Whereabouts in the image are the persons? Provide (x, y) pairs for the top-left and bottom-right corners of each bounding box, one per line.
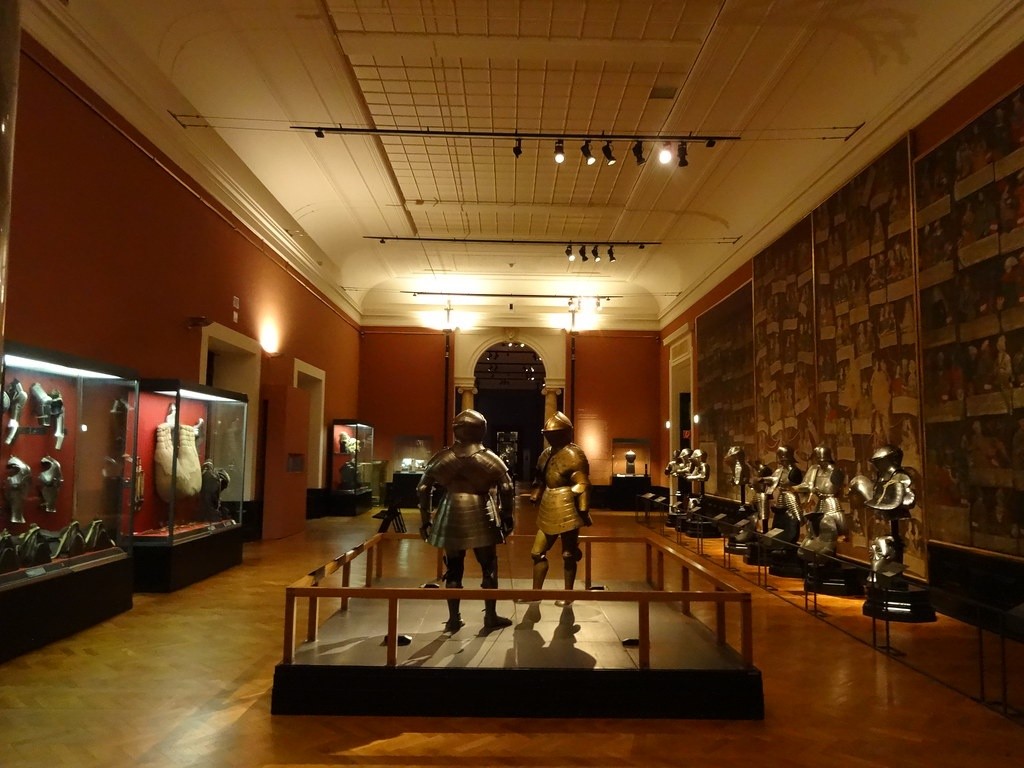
(725, 446), (750, 484)
(677, 448), (695, 480)
(686, 449), (710, 481)
(514, 413), (592, 603)
(666, 450), (683, 475)
(415, 410), (517, 634)
(769, 447), (804, 510)
(865, 444), (917, 514)
(793, 445), (849, 555)
